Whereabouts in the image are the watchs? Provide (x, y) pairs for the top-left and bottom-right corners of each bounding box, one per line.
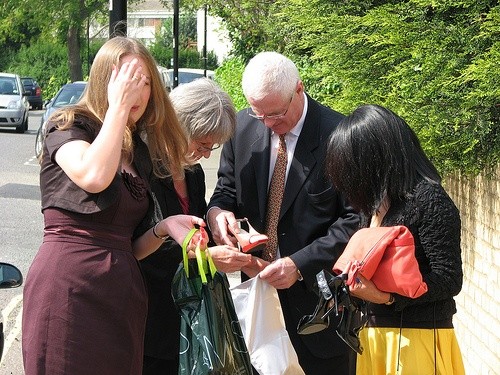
(385, 293), (394, 306)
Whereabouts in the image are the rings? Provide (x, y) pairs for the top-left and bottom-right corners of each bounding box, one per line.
(133, 76), (139, 82)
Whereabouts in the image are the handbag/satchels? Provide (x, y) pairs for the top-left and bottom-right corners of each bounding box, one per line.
(332, 226), (428, 298)
(229, 274), (307, 375)
(170, 227), (253, 375)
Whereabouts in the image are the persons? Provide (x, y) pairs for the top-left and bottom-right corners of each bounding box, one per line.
(21, 35), (209, 375)
(202, 52), (357, 375)
(139, 78), (270, 375)
(325, 104), (465, 375)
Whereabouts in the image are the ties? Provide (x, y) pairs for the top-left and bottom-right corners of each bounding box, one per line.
(261, 132), (287, 263)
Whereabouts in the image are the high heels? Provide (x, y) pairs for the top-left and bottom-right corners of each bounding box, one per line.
(321, 285), (366, 356)
(296, 269), (342, 335)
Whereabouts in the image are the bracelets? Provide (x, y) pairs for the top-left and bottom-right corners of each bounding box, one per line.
(152, 222), (169, 240)
(296, 268), (302, 277)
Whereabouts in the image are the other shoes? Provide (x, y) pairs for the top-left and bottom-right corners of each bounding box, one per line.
(227, 218), (267, 253)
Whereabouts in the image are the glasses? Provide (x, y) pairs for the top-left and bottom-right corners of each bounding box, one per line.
(193, 140), (221, 151)
(247, 88), (295, 119)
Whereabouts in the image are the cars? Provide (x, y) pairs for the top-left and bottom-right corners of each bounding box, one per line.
(0, 72), (30, 133)
(34, 81), (88, 160)
(164, 66), (215, 92)
(20, 76), (43, 109)
